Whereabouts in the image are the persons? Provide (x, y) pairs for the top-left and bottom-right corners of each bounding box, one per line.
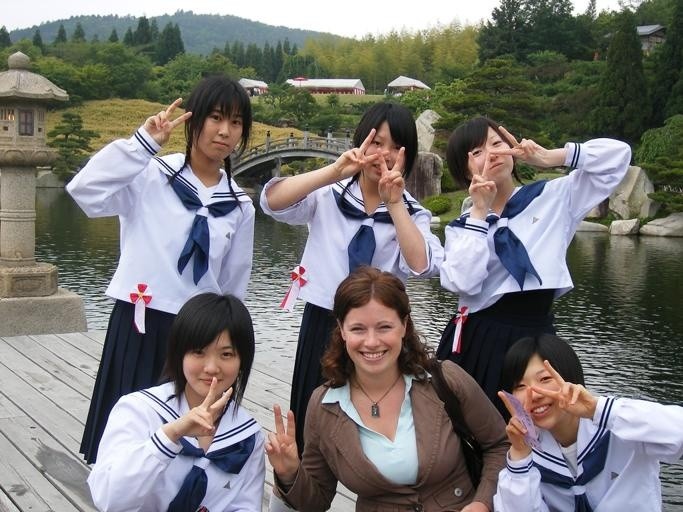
(490, 332), (683, 512)
(262, 264), (511, 512)
(430, 116), (635, 422)
(256, 100), (447, 512)
(62, 75), (256, 464)
(85, 291), (266, 512)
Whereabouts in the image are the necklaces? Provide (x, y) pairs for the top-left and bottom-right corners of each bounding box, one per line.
(352, 372), (402, 419)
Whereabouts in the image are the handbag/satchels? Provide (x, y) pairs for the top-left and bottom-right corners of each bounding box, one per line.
(444, 398), (483, 488)
